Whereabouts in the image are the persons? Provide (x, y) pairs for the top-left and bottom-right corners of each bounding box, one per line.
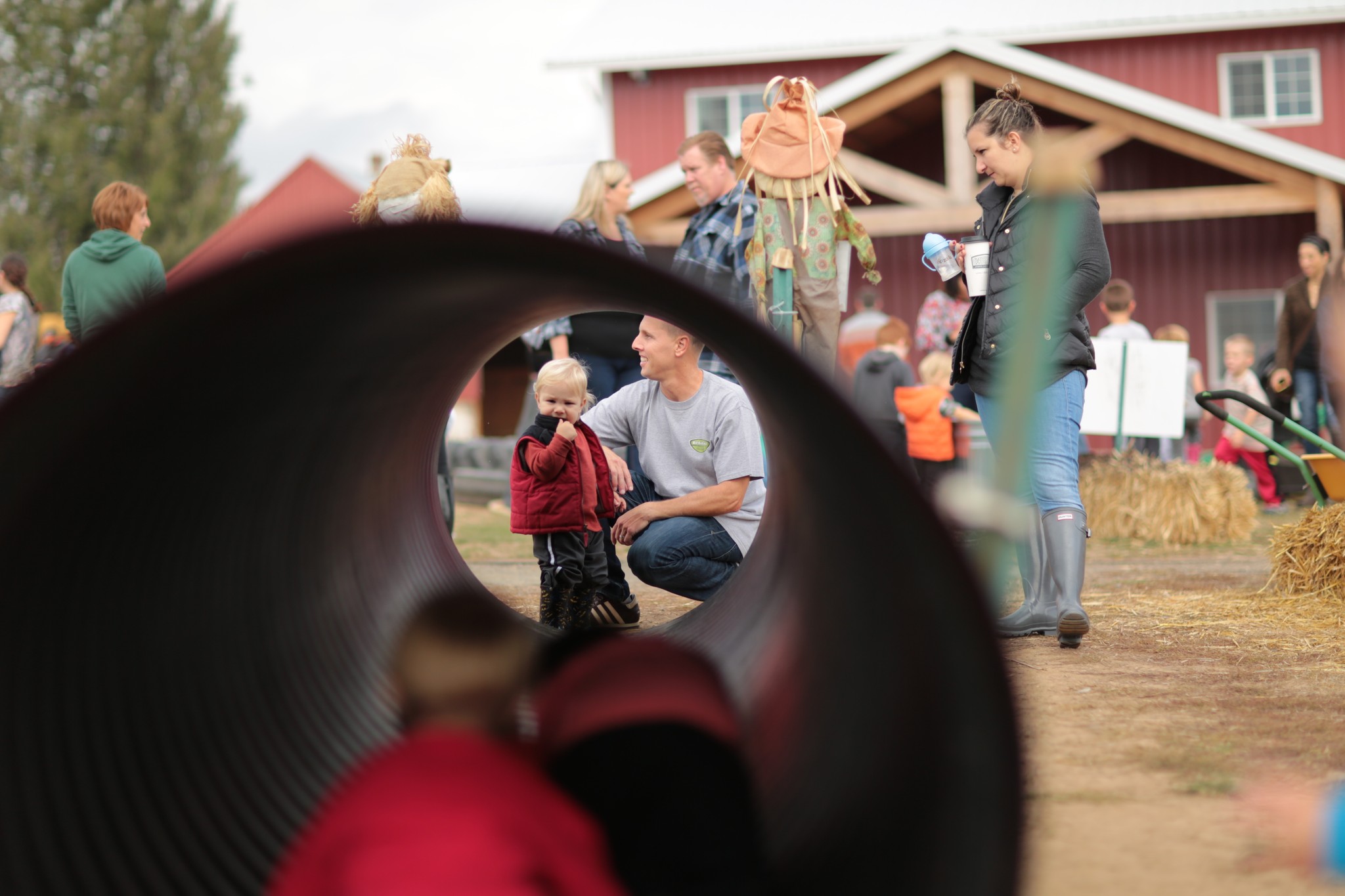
(731, 73), (885, 383)
(578, 309), (770, 632)
(59, 182), (171, 352)
(665, 134), (764, 316)
(532, 156), (659, 412)
(938, 72), (1112, 648)
(921, 266), (973, 365)
(1090, 273), (1151, 463)
(265, 578), (629, 896)
(507, 356), (626, 634)
(1267, 234), (1344, 502)
(1151, 323), (1216, 447)
(1, 252), (40, 406)
(890, 353), (987, 489)
(1205, 332), (1280, 515)
(850, 315), (915, 466)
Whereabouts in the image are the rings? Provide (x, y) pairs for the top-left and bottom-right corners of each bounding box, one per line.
(619, 532), (627, 538)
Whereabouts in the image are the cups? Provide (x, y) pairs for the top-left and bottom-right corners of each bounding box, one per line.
(961, 236), (994, 299)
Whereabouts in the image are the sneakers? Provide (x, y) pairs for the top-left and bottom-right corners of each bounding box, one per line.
(586, 593), (640, 627)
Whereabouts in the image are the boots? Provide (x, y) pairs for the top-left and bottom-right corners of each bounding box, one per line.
(990, 506), (1060, 636)
(1041, 506), (1091, 648)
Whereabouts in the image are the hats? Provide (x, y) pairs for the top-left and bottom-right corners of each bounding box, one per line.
(740, 76), (848, 180)
(893, 386), (945, 421)
(79, 227), (139, 262)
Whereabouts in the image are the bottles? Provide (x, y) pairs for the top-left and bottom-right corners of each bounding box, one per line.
(920, 232), (961, 282)
